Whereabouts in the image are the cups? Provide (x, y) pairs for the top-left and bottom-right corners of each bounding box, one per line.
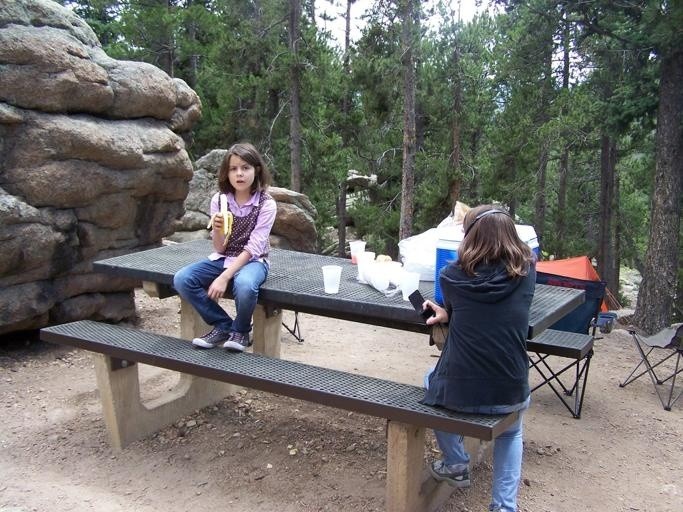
(400, 272), (419, 300)
(347, 239), (366, 265)
(357, 252), (408, 297)
(321, 265), (343, 294)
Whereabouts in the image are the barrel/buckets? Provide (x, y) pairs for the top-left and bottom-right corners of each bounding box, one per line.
(598, 315), (614, 334)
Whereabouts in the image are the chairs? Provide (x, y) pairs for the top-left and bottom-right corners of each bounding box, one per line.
(618, 319), (683, 411)
(530, 267), (608, 420)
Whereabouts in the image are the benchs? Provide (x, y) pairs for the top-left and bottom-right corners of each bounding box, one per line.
(40, 318), (519, 511)
(252, 301), (594, 419)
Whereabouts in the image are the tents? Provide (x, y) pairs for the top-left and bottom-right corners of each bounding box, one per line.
(528, 253), (609, 315)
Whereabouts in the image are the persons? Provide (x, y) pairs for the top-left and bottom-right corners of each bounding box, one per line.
(415, 205), (539, 512)
(173, 142), (279, 351)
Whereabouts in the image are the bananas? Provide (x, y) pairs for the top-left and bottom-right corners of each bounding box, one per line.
(206, 194), (233, 247)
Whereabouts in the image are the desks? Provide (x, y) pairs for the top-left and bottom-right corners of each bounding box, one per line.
(91, 239), (584, 451)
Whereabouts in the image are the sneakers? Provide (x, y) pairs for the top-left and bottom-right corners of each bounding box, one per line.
(223, 332), (248, 352)
(431, 460), (470, 488)
(193, 328), (227, 349)
